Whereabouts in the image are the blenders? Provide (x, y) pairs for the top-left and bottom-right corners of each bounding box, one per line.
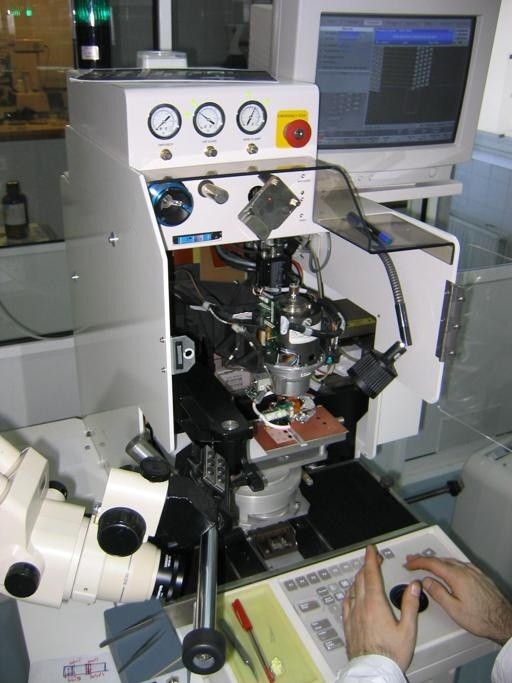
(10, 39), (50, 114)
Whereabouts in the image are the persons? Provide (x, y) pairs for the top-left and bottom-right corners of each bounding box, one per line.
(335, 542), (512, 683)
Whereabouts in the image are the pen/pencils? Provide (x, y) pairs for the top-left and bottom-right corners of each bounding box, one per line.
(231, 598), (275, 683)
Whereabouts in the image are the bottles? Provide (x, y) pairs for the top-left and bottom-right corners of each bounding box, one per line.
(74, 0), (111, 68)
(1, 180), (29, 241)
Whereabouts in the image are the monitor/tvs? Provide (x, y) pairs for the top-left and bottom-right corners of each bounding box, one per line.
(247, 0), (503, 191)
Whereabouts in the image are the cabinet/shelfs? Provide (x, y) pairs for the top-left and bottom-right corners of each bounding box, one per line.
(0, 0), (172, 363)
